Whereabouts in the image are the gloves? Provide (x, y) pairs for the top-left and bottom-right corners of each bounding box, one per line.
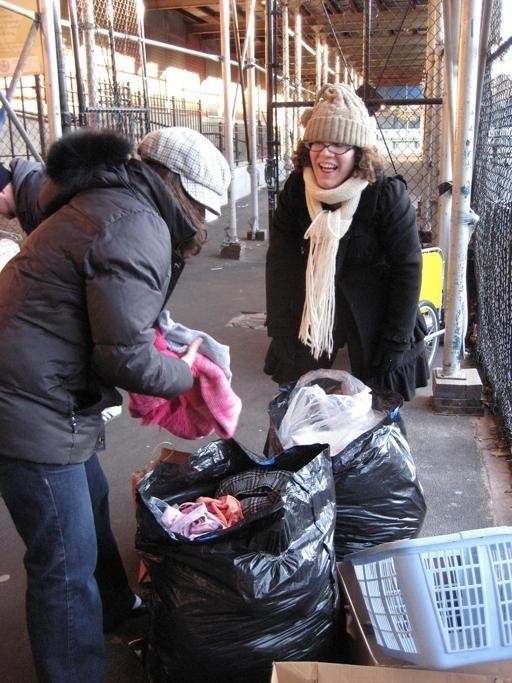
(372, 344), (403, 374)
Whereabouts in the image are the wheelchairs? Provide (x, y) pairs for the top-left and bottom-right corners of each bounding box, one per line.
(416, 248), (444, 367)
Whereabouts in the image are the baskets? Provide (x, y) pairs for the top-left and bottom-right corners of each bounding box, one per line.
(343, 525), (512, 670)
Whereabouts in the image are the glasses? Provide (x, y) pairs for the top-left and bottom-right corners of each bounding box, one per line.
(308, 141), (354, 155)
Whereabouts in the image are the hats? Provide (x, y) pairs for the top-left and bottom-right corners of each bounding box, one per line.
(301, 82), (371, 153)
(136, 126), (231, 217)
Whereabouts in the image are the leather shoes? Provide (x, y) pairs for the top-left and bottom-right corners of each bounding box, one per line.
(104, 592), (144, 621)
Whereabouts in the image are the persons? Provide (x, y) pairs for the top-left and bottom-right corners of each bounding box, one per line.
(260, 82), (432, 417)
(2, 154), (60, 239)
(1, 123), (235, 682)
